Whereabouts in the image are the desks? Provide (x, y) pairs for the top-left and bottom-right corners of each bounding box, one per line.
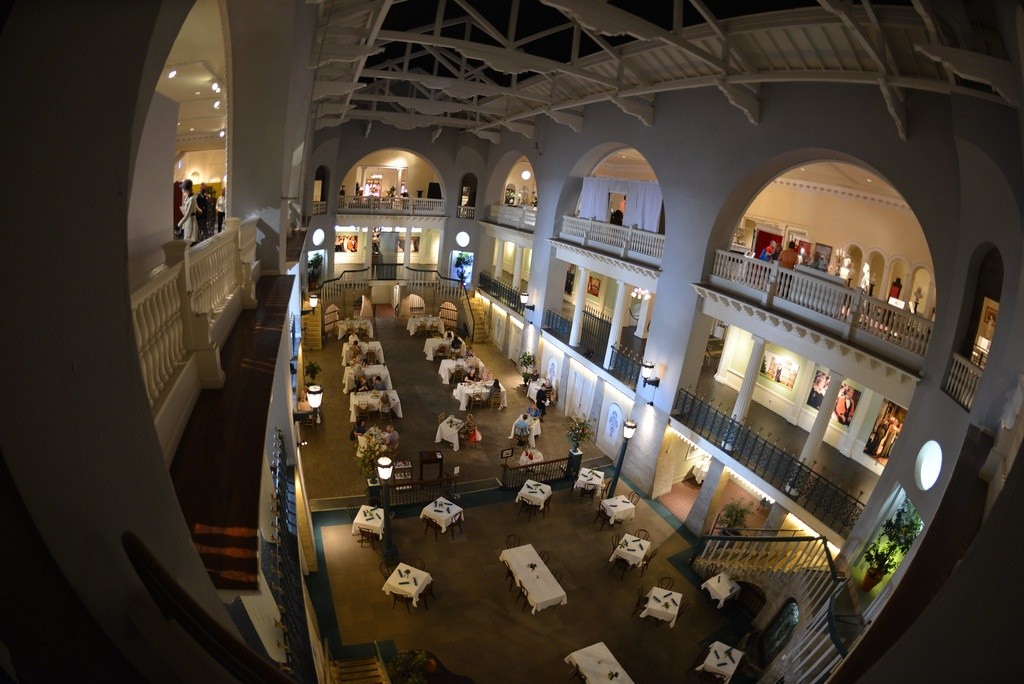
(565, 642), (634, 684)
(639, 587), (683, 629)
(499, 544), (567, 615)
(526, 382), (550, 406)
(456, 381), (507, 411)
(438, 357), (485, 385)
(338, 320), (373, 340)
(573, 468), (604, 498)
(695, 641), (745, 684)
(701, 571), (742, 609)
(516, 479), (552, 511)
(508, 414), (541, 448)
(407, 317), (444, 336)
(352, 505), (384, 540)
(597, 495), (635, 524)
(423, 337), (466, 361)
(519, 449), (544, 471)
(382, 563), (432, 608)
(435, 415), (482, 451)
(692, 460), (709, 487)
(609, 533), (651, 571)
(420, 496), (464, 533)
(342, 341), (402, 457)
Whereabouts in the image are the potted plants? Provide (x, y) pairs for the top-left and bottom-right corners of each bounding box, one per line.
(862, 495), (924, 591)
(455, 252), (474, 289)
(303, 361), (323, 394)
(518, 352), (535, 382)
(719, 500), (756, 538)
(308, 253), (324, 290)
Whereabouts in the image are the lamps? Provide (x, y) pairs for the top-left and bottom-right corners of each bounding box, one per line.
(520, 293), (535, 312)
(977, 336), (992, 367)
(292, 384), (323, 425)
(641, 361), (660, 388)
(630, 288), (651, 301)
(295, 439), (308, 448)
(301, 294), (318, 316)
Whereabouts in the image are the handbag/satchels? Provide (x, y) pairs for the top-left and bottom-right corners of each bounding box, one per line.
(475, 428), (482, 441)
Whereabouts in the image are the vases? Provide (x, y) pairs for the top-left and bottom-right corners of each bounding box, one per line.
(572, 442), (580, 452)
(370, 470), (377, 484)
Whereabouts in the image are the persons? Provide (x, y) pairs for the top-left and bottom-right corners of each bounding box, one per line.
(463, 367), (476, 383)
(382, 425), (400, 450)
(444, 327), (477, 357)
(765, 239), (798, 299)
(300, 386), (308, 402)
(775, 364), (782, 382)
(350, 419), (365, 441)
(515, 370), (548, 442)
(344, 330), (386, 391)
(484, 378), (500, 408)
(835, 383), (858, 426)
(177, 180), (225, 248)
(865, 414), (901, 458)
(531, 197), (538, 207)
(459, 413), (475, 439)
(334, 236), (358, 252)
(807, 370), (830, 410)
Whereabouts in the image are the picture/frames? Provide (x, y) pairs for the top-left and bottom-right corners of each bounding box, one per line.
(984, 307), (1000, 327)
(814, 241), (833, 271)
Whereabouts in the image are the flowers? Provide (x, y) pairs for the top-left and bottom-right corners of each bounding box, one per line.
(353, 435), (388, 479)
(564, 413), (597, 444)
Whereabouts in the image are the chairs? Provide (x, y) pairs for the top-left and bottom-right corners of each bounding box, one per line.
(346, 464), (747, 684)
(347, 319), (391, 426)
(417, 321), (554, 447)
(388, 438), (401, 460)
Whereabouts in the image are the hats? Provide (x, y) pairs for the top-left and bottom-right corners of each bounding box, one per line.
(179, 179), (193, 191)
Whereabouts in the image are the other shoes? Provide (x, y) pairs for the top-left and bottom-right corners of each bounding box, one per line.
(540, 418), (544, 423)
